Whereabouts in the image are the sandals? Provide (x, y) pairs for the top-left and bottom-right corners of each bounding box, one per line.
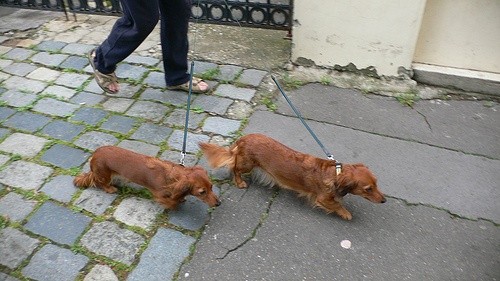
(89, 48), (120, 94)
(167, 76), (209, 93)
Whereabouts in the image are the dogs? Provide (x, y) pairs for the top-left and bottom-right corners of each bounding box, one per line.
(74, 145), (222, 211)
(198, 133), (386, 221)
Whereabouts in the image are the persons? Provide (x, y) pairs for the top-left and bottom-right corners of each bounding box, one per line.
(87, 0), (210, 94)
